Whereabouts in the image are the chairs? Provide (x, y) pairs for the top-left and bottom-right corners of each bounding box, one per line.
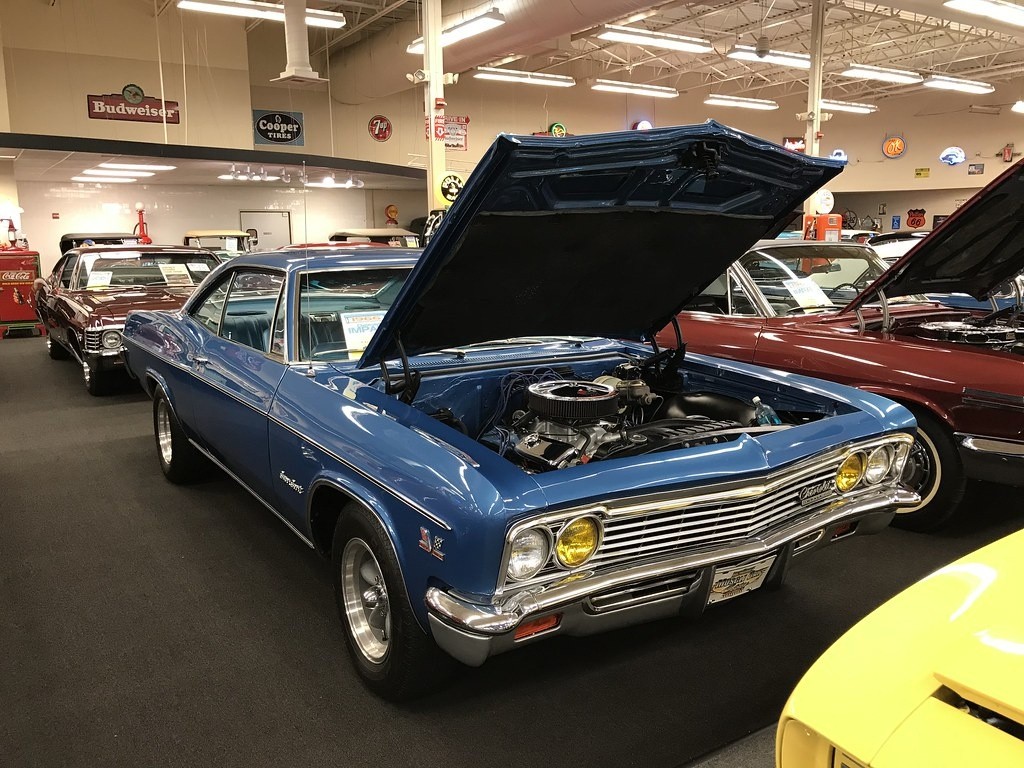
(262, 320), (319, 359)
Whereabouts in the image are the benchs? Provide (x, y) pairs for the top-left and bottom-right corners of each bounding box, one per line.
(223, 304), (389, 360)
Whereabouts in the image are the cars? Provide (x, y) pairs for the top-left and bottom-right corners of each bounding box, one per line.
(119, 120), (923, 710)
(776, 530), (1024, 768)
(32, 228), (1020, 398)
(649, 159), (1024, 533)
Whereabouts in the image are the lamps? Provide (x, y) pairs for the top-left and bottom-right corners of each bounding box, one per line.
(279, 168), (291, 183)
(245, 167), (255, 180)
(472, 66), (577, 88)
(406, 68), (430, 84)
(968, 104), (1001, 115)
(257, 165), (268, 182)
(345, 176), (364, 188)
(405, 8), (505, 54)
(838, 62), (924, 85)
(726, 45), (812, 68)
(443, 73), (459, 85)
(298, 172), (307, 184)
(924, 75), (996, 95)
(943, 0), (1024, 27)
(175, 0), (346, 29)
(230, 164), (241, 179)
(821, 98), (880, 114)
(703, 94), (779, 110)
(588, 77), (679, 99)
(598, 24), (715, 54)
